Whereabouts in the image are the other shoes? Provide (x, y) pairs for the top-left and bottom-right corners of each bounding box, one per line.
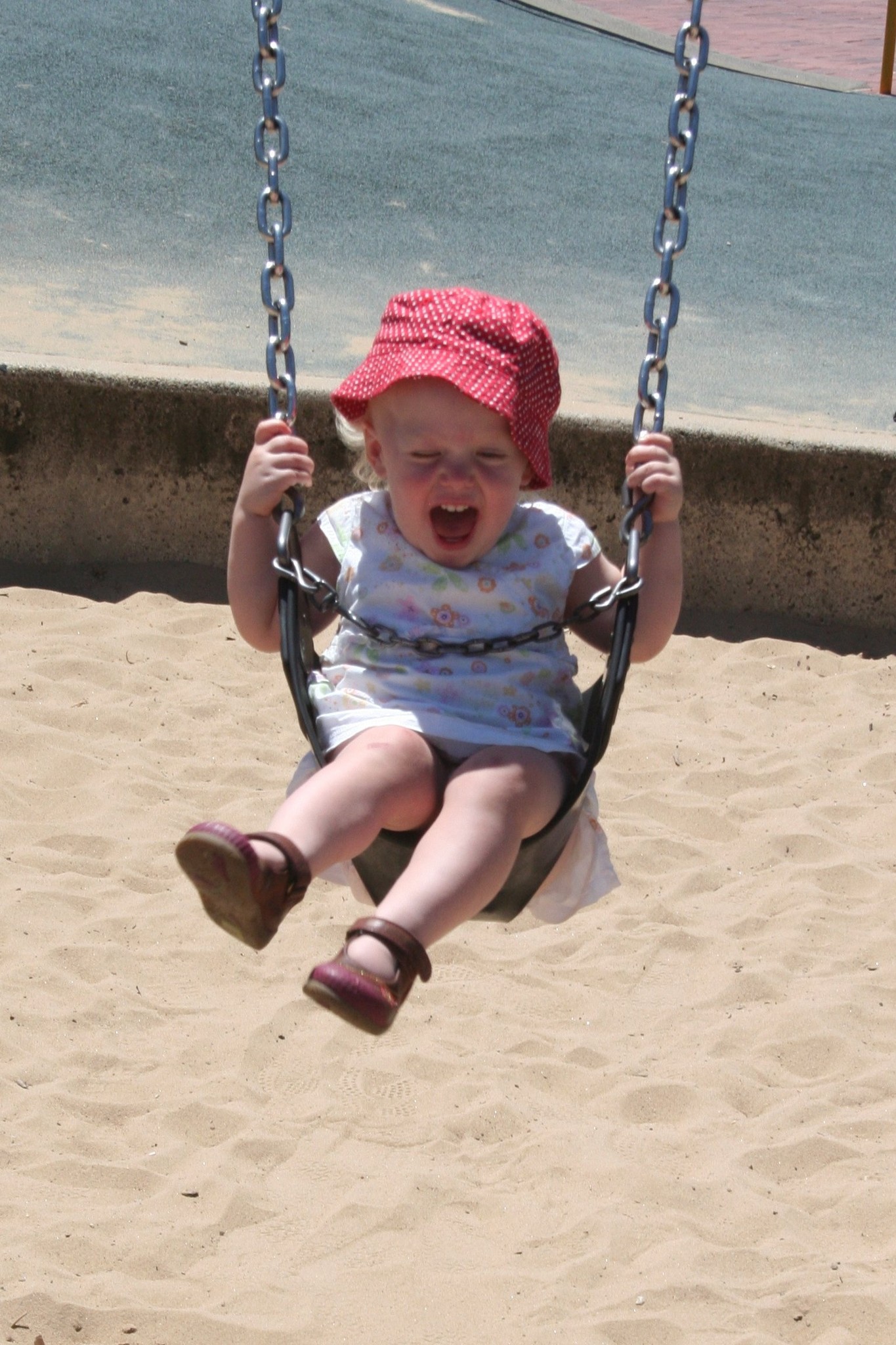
(303, 917), (431, 1036)
(177, 822), (311, 948)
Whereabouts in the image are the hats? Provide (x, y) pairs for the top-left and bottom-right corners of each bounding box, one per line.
(331, 289), (561, 491)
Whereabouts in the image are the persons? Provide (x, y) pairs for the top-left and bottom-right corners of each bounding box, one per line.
(173, 280), (695, 1035)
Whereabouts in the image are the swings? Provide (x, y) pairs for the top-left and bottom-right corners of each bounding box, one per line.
(250, 0), (710, 924)
